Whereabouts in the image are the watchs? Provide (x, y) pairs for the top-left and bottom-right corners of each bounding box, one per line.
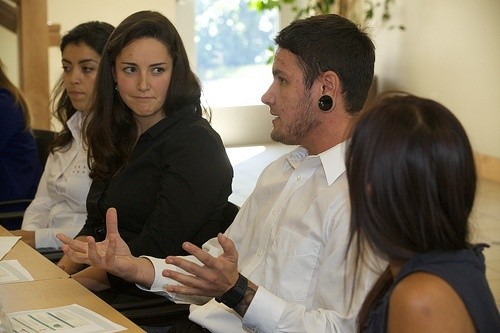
(215, 273), (248, 308)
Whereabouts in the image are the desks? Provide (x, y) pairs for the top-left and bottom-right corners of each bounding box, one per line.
(0, 225), (148, 333)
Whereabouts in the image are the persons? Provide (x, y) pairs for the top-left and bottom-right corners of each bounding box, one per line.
(56, 14), (375, 333)
(56, 10), (233, 333)
(10, 22), (115, 250)
(0, 60), (39, 212)
(345, 96), (500, 333)
